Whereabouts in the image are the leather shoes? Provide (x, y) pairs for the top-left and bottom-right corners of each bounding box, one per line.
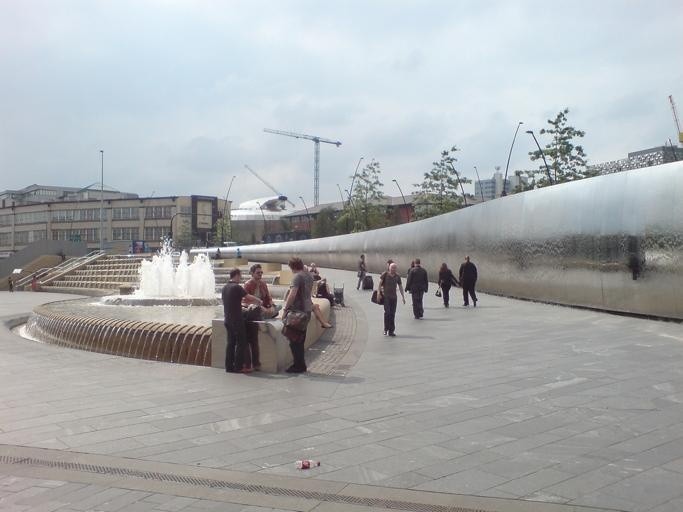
(384, 331), (387, 335)
(473, 299), (477, 307)
(463, 302), (469, 306)
(322, 324), (333, 328)
(286, 364), (306, 372)
(389, 333), (396, 336)
(226, 367), (261, 373)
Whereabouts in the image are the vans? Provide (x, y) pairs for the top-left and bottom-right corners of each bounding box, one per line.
(0, 252), (15, 258)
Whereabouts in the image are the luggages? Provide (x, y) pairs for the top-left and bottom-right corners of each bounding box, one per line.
(362, 276), (373, 290)
(333, 283), (344, 304)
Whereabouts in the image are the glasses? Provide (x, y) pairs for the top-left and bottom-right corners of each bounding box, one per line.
(257, 272), (263, 274)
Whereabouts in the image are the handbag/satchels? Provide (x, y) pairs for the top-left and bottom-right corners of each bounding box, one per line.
(357, 271), (365, 277)
(435, 290), (441, 297)
(371, 290), (385, 305)
(282, 309), (309, 333)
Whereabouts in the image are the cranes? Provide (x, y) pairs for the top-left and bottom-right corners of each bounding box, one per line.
(261, 127), (344, 204)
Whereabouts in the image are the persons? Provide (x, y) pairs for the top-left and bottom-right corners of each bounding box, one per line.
(385, 260), (393, 271)
(242, 263), (283, 320)
(8, 277), (13, 292)
(375, 262), (406, 338)
(213, 248), (223, 259)
(315, 278), (335, 306)
(308, 262), (321, 281)
(127, 244), (131, 253)
(457, 254), (478, 309)
(403, 257), (428, 321)
(284, 285), (333, 331)
(220, 267), (263, 373)
(31, 275), (37, 291)
(271, 275), (280, 285)
(355, 254), (368, 290)
(235, 248), (241, 259)
(437, 262), (458, 309)
(406, 260), (414, 277)
(279, 256), (313, 376)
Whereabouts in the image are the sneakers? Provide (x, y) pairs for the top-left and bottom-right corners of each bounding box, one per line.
(275, 305), (282, 311)
(415, 316), (424, 320)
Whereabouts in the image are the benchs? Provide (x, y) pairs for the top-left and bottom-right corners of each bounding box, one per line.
(211, 294), (330, 374)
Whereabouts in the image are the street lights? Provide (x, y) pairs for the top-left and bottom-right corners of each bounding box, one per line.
(444, 161), (467, 207)
(170, 212), (188, 247)
(99, 149), (105, 251)
(524, 129), (553, 186)
(221, 157), (369, 242)
(500, 120), (525, 197)
(391, 179), (410, 225)
(473, 166), (484, 202)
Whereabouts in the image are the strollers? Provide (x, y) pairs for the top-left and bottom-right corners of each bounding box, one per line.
(331, 283), (347, 308)
